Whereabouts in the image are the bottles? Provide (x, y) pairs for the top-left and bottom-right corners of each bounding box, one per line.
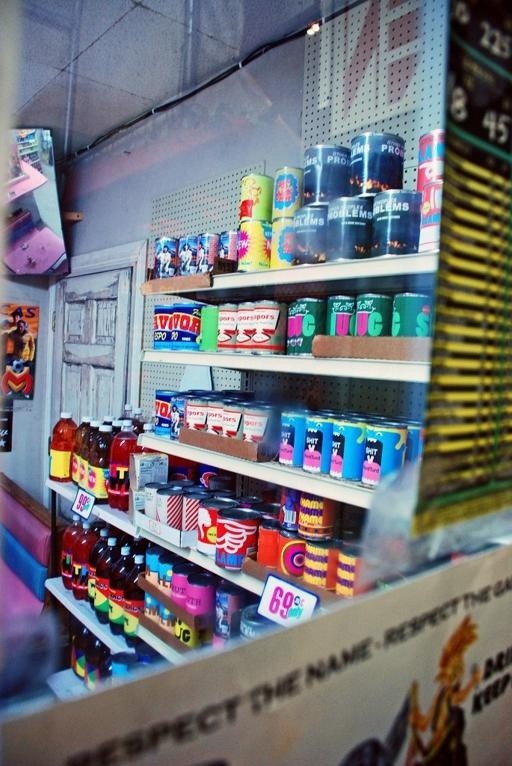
(61, 514), (83, 590)
(70, 624), (92, 678)
(123, 555), (145, 647)
(87, 527), (112, 610)
(94, 536), (122, 624)
(84, 638), (111, 693)
(49, 403), (154, 512)
(108, 546), (134, 635)
(71, 521), (96, 601)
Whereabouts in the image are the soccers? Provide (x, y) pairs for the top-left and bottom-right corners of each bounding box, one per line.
(11, 360), (24, 374)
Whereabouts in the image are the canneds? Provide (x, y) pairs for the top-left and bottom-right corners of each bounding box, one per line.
(155, 389), (314, 458)
(154, 236), (178, 278)
(237, 220), (273, 272)
(111, 642), (165, 676)
(418, 179), (443, 253)
(302, 145), (351, 205)
(128, 489), (145, 523)
(130, 451), (168, 492)
(150, 291), (434, 357)
(218, 231), (237, 262)
(144, 475), (361, 598)
(279, 408), (422, 486)
(291, 207), (328, 265)
(417, 129), (444, 191)
(347, 132), (405, 198)
(196, 232), (219, 273)
(271, 166), (304, 222)
(176, 235), (198, 276)
(325, 197), (373, 261)
(270, 219), (295, 269)
(238, 172), (274, 226)
(144, 543), (276, 649)
(371, 189), (422, 258)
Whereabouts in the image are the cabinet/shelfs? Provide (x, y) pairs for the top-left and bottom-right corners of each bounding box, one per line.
(15, 128), (41, 174)
(38, 413), (408, 700)
(136, 247), (438, 511)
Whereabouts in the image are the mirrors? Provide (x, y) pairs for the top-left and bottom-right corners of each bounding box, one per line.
(0, 125), (69, 277)
